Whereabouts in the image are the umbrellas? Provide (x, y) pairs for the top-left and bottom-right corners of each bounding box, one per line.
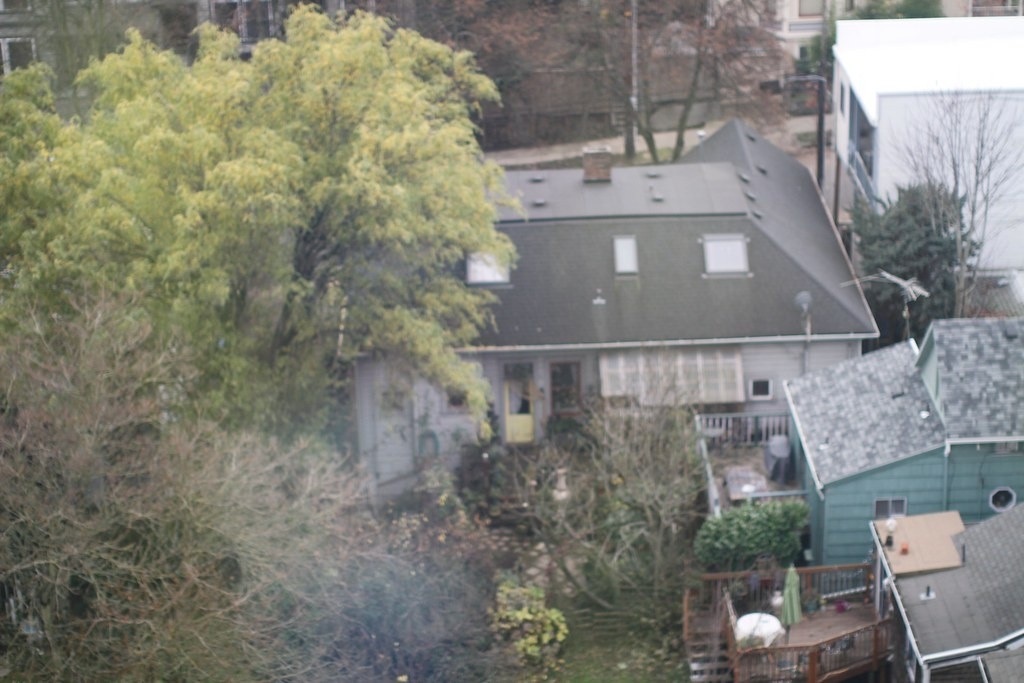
(783, 563), (801, 642)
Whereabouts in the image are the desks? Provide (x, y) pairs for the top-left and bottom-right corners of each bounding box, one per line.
(764, 435), (796, 485)
(723, 464), (768, 501)
(736, 612), (785, 650)
(701, 426), (724, 448)
